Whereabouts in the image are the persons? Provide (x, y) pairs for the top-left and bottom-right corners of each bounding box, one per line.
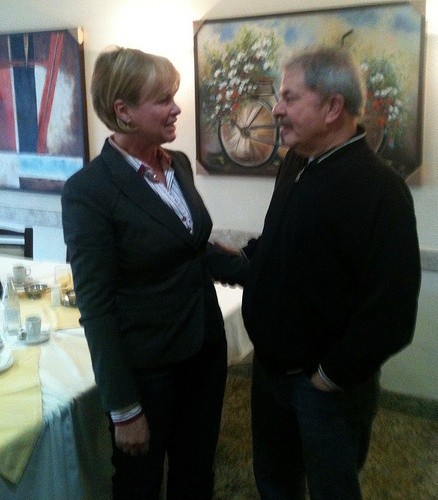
(213, 50), (422, 500)
(62, 48), (251, 500)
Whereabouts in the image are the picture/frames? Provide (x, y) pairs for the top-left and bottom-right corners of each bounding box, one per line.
(193, 0), (427, 188)
(0, 26), (91, 196)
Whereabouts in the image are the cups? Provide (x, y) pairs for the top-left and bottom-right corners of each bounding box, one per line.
(55, 267), (73, 291)
(25, 316), (41, 338)
(51, 285), (61, 306)
(13, 266), (31, 283)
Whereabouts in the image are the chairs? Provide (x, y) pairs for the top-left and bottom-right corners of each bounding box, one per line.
(0, 227), (34, 260)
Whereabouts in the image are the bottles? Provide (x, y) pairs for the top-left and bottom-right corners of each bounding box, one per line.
(5, 276), (21, 336)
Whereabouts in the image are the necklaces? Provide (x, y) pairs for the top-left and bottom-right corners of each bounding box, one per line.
(153, 162), (159, 179)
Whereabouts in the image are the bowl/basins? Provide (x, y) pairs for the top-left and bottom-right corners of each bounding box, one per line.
(25, 284), (47, 299)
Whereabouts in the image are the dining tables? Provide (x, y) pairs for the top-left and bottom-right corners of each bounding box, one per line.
(0, 255), (258, 500)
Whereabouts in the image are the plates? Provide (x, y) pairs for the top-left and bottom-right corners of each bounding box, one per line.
(22, 332), (49, 344)
(14, 279), (34, 287)
